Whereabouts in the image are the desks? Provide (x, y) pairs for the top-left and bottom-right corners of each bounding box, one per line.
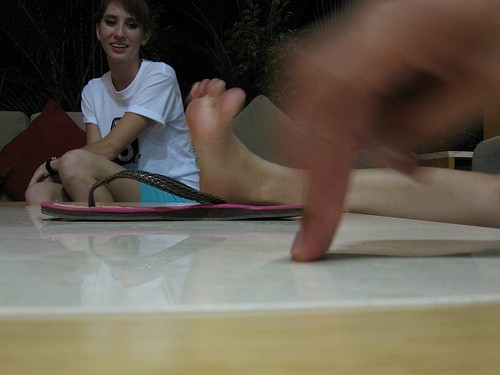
(0, 201), (500, 375)
(415, 150), (475, 170)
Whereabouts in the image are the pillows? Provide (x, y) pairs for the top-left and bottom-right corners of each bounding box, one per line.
(232, 95), (313, 169)
(1, 100), (86, 221)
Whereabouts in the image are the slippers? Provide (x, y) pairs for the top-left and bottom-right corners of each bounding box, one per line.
(40, 170), (305, 220)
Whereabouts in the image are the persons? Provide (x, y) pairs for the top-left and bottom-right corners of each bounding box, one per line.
(188, 75), (500, 230)
(260, 1), (499, 261)
(24, 0), (200, 208)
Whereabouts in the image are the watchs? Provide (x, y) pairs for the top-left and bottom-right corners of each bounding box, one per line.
(45, 156), (56, 174)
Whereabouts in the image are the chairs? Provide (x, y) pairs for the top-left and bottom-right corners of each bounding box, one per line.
(0, 111), (85, 152)
